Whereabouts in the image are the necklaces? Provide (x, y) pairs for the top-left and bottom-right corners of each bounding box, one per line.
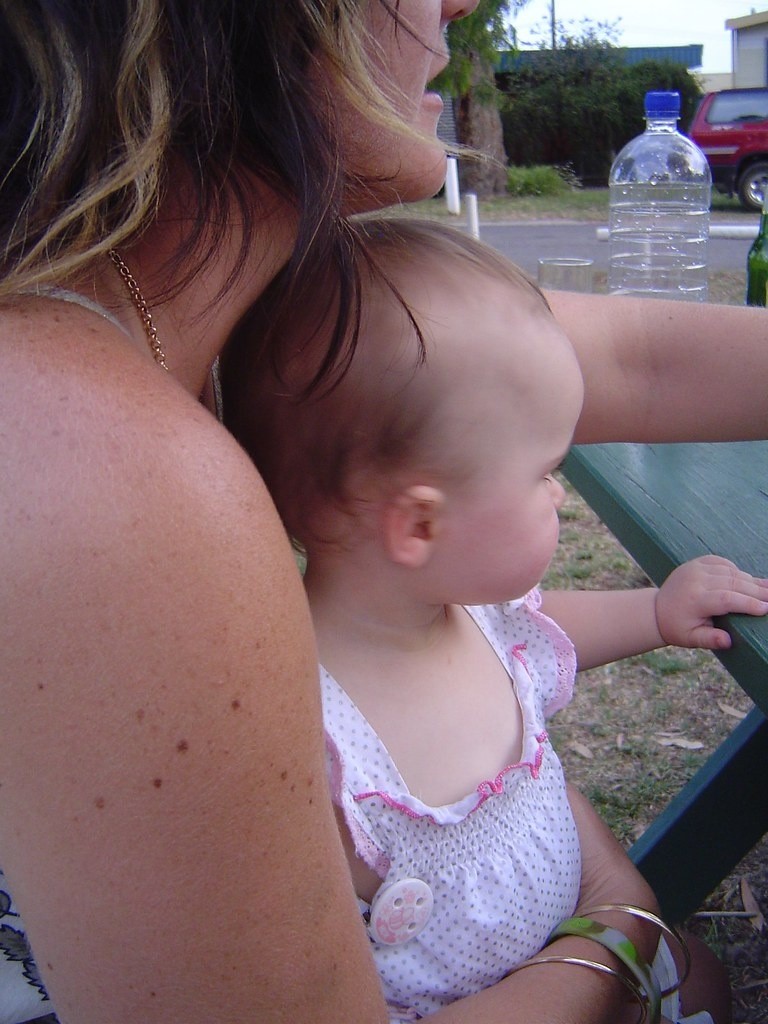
(93, 209), (165, 372)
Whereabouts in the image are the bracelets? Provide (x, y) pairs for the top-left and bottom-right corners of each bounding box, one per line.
(505, 956), (646, 1024)
(547, 917), (662, 1023)
(574, 905), (690, 998)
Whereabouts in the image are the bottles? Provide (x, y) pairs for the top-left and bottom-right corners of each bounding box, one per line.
(608, 92), (712, 304)
(747, 184), (768, 308)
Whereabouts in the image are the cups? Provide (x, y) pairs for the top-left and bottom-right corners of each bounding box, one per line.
(538, 258), (594, 294)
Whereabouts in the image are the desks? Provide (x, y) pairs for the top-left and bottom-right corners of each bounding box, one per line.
(508, 443), (768, 956)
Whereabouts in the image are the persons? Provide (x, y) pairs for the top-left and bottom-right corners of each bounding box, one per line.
(220, 222), (768, 1024)
(0, 3), (768, 1023)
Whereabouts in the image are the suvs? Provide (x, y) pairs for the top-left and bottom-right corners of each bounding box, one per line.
(689, 87), (768, 212)
